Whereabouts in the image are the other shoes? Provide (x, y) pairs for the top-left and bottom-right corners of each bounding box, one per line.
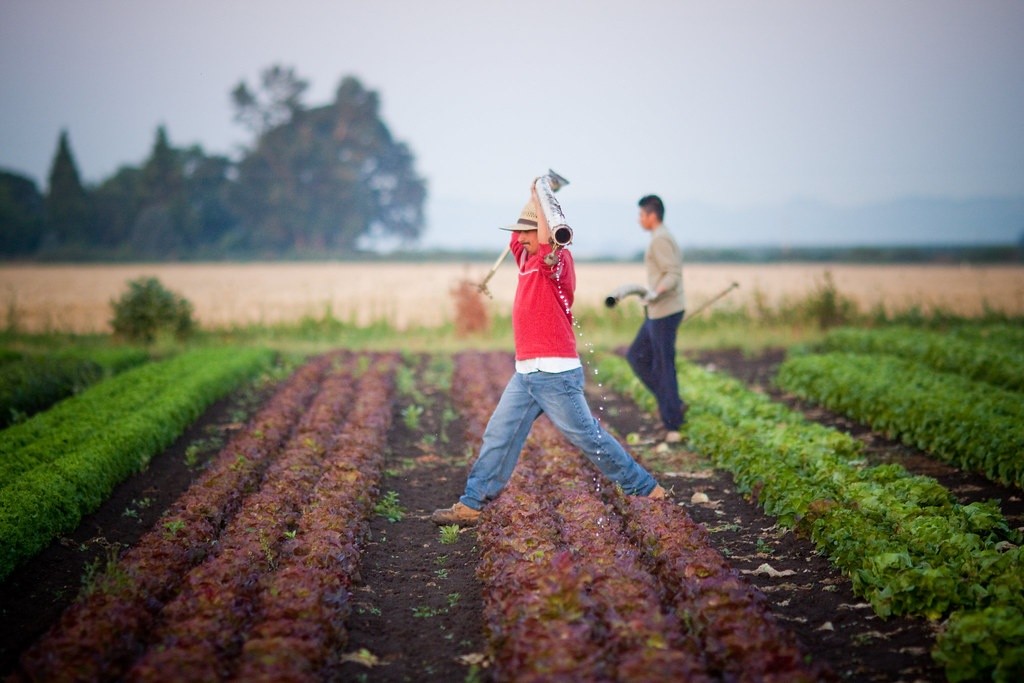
(649, 438), (689, 453)
(654, 404), (689, 433)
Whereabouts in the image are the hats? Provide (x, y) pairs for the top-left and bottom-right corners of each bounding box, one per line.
(499, 198), (538, 230)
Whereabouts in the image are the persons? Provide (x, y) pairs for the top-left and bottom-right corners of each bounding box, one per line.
(626, 196), (689, 448)
(433, 169), (665, 525)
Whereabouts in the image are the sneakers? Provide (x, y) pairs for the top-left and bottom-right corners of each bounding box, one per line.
(649, 485), (669, 501)
(431, 503), (482, 526)
